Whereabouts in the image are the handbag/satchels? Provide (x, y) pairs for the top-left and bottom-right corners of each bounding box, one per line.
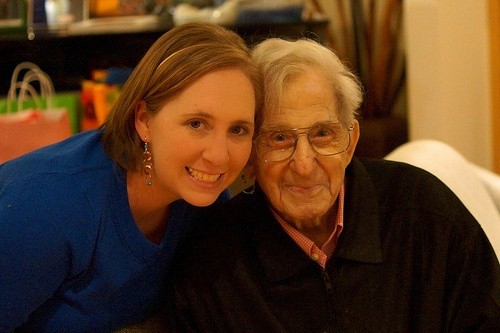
(1, 74), (71, 168)
(1, 61), (80, 143)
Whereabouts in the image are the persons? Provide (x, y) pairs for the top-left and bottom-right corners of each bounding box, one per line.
(0, 20), (266, 333)
(163, 37), (500, 333)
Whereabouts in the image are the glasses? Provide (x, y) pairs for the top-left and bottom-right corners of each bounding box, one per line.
(254, 121), (355, 163)
